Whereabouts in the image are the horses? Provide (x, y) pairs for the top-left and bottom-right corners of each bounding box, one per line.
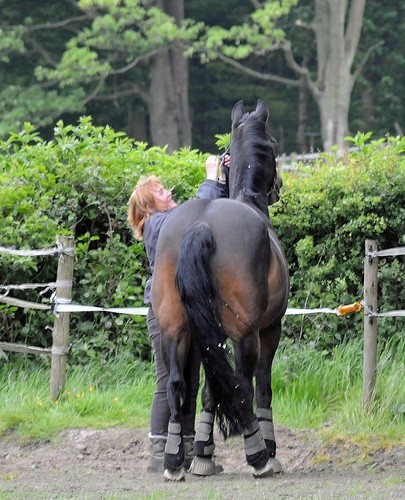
(149, 98), (290, 481)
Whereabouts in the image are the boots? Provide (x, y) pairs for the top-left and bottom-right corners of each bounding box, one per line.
(146, 432), (168, 472)
(182, 435), (195, 471)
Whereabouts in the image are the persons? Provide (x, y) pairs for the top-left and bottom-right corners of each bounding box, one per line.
(127, 155), (231, 472)
